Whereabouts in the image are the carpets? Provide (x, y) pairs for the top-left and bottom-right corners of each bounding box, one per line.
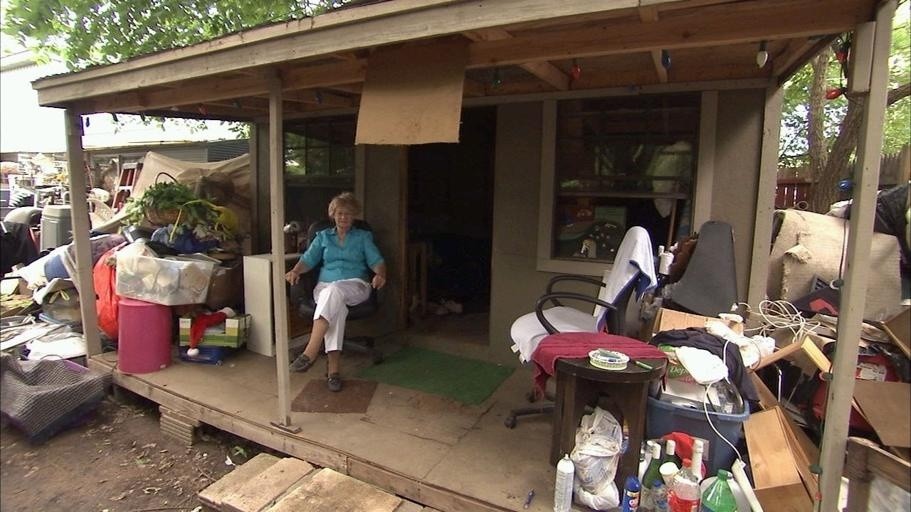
(291, 379), (379, 414)
(359, 346), (516, 405)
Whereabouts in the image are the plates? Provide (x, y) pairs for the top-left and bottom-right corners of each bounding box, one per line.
(589, 350), (630, 370)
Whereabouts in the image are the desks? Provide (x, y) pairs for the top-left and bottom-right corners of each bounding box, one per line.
(538, 332), (668, 485)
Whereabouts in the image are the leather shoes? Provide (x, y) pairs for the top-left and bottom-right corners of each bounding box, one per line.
(327, 372), (340, 391)
(289, 354), (315, 372)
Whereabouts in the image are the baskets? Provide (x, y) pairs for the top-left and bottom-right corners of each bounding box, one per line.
(144, 172), (187, 224)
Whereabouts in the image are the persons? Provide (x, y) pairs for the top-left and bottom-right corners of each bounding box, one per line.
(284, 192), (387, 392)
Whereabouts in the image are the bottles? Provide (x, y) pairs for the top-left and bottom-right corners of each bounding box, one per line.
(620, 440), (737, 512)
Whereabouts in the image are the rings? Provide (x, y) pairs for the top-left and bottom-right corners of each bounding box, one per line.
(378, 279), (382, 282)
(287, 273), (290, 276)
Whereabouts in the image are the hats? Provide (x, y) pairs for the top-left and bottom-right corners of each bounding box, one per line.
(187, 307), (236, 358)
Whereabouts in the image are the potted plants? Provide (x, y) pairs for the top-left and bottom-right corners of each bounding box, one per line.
(121, 171), (201, 227)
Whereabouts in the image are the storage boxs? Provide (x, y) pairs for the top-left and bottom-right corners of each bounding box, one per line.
(649, 377), (752, 475)
(743, 404), (817, 512)
(809, 350), (901, 434)
(115, 249), (255, 364)
(1, 359), (101, 446)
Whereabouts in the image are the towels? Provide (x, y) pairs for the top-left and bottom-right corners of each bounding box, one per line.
(509, 223), (658, 362)
(528, 324), (672, 414)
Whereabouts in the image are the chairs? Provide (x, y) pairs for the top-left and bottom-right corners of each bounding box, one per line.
(292, 218), (378, 377)
(505, 226), (651, 428)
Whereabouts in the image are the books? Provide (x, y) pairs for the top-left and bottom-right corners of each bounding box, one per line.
(805, 312), (892, 344)
(800, 322), (870, 350)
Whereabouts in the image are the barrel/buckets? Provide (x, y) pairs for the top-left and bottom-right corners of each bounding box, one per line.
(116, 298), (174, 376)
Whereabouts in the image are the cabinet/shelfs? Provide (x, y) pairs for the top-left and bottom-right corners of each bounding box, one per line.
(243, 253), (321, 356)
